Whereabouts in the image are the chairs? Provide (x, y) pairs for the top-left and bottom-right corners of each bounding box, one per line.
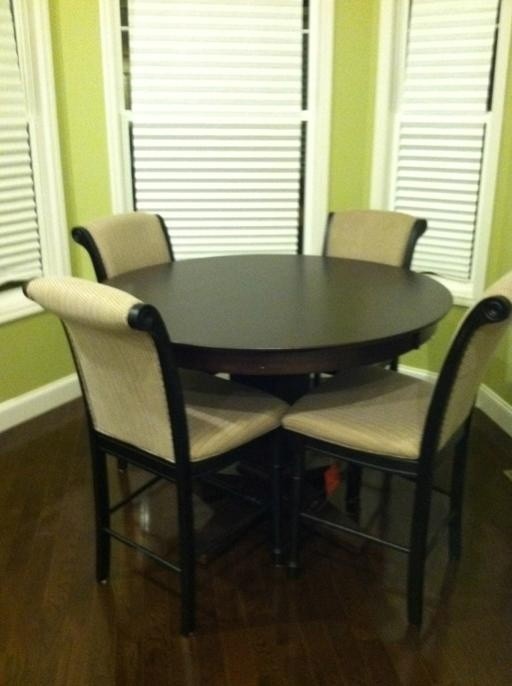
(320, 211), (425, 269)
(22, 273), (284, 628)
(280, 265), (512, 626)
(67, 210), (176, 286)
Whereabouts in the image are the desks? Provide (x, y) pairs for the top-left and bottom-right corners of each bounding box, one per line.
(99, 253), (456, 555)
